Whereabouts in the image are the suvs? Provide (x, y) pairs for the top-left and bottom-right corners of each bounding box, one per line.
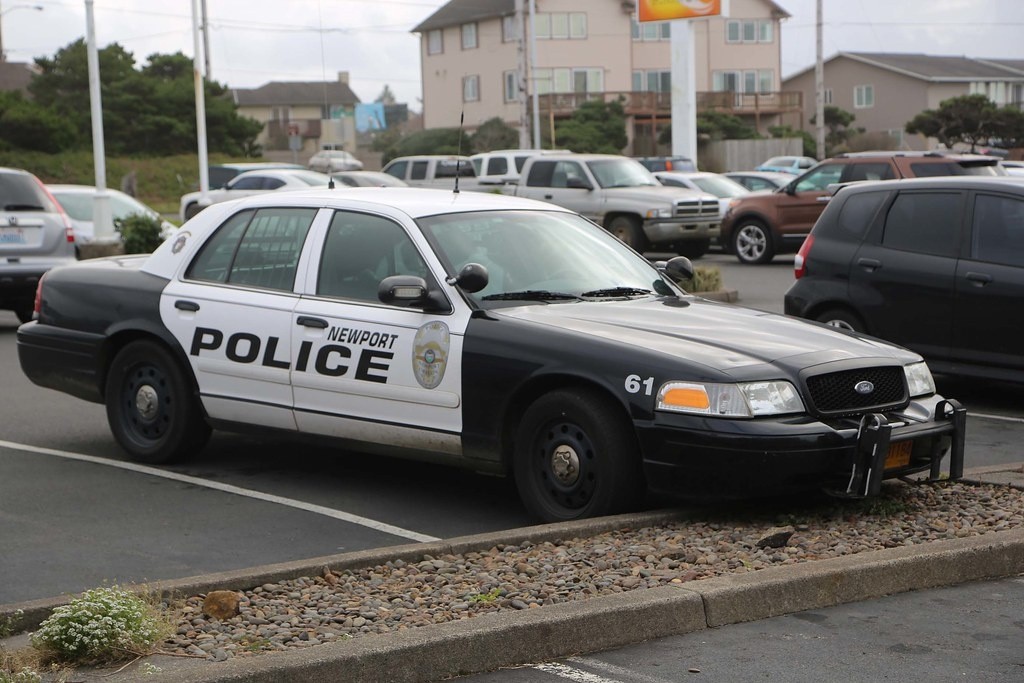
(0, 166), (84, 324)
(721, 151), (1007, 264)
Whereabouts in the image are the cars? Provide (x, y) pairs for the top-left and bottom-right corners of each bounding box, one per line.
(309, 149), (364, 176)
(16, 187), (969, 525)
(650, 169), (752, 223)
(780, 176), (1024, 407)
(636, 156), (696, 177)
(722, 171), (813, 194)
(334, 171), (410, 191)
(45, 182), (181, 261)
(752, 155), (820, 178)
(180, 166), (349, 226)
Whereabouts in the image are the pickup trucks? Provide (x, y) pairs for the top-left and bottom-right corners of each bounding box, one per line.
(463, 152), (742, 255)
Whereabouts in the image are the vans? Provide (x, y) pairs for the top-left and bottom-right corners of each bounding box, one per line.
(469, 148), (571, 183)
(380, 153), (481, 193)
